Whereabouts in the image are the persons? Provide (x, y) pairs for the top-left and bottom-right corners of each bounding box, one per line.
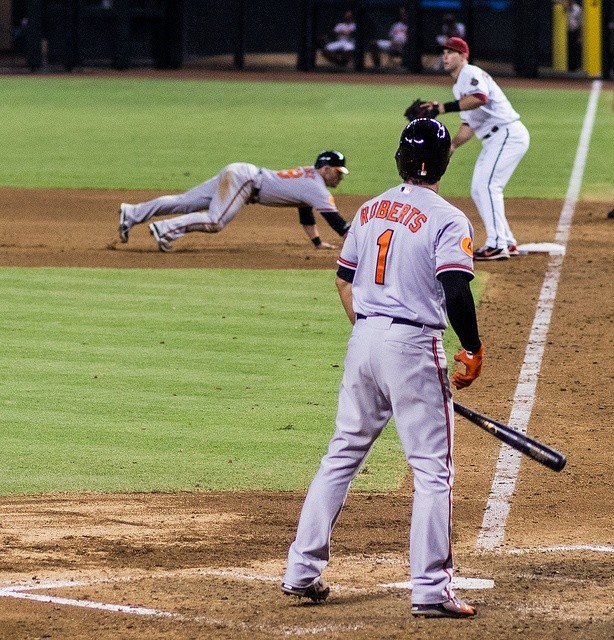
(418, 13), (467, 75)
(565, 0), (584, 65)
(1, 17), (40, 67)
(366, 4), (416, 72)
(278, 116), (485, 620)
(417, 36), (531, 261)
(321, 9), (360, 71)
(116, 148), (352, 254)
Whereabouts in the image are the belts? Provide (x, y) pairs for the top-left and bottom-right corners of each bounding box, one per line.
(484, 126), (498, 138)
(357, 313), (423, 328)
(249, 168), (262, 203)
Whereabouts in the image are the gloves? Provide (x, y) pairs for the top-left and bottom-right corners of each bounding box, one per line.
(452, 343), (484, 390)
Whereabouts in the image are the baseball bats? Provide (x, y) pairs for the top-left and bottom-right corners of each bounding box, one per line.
(452, 402), (566, 472)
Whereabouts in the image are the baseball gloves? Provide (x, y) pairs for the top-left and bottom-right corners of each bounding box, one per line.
(404, 98), (439, 122)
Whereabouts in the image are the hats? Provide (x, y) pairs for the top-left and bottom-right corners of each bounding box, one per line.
(315, 150), (350, 174)
(441, 37), (469, 58)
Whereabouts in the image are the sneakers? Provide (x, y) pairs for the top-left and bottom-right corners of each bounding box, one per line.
(281, 580), (330, 601)
(474, 245), (519, 256)
(117, 203), (135, 243)
(473, 248), (511, 261)
(410, 593), (478, 617)
(149, 220), (172, 252)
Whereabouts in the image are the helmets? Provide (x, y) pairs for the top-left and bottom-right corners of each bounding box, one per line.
(395, 117), (450, 183)
